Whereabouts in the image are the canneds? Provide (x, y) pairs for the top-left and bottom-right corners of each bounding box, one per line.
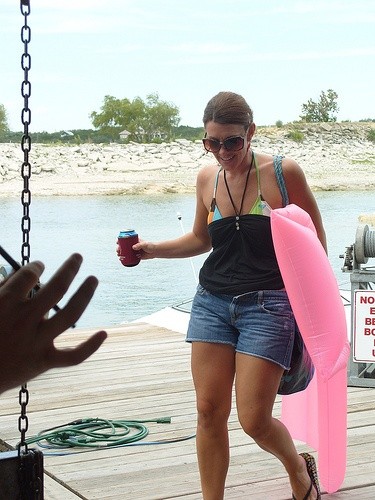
(117, 229), (140, 267)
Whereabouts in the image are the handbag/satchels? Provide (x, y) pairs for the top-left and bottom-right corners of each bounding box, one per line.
(278, 319), (315, 395)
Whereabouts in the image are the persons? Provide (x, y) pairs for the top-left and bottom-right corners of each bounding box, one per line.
(117, 92), (328, 500)
(0, 253), (107, 395)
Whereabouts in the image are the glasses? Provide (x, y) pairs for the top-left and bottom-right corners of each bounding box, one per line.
(202, 127), (249, 152)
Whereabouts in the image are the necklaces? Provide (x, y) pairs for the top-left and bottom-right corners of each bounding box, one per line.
(222, 150), (255, 231)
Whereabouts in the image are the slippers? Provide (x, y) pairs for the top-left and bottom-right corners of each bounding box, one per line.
(291, 452), (322, 500)
